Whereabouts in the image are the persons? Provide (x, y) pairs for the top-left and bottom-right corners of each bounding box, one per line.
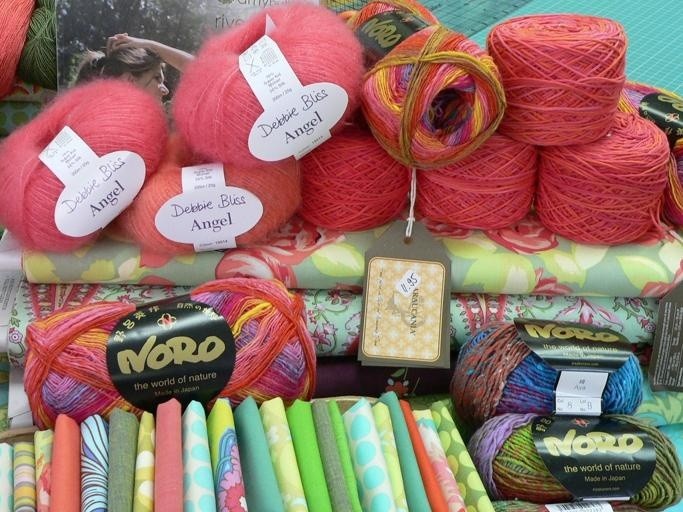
(68, 32), (200, 129)
